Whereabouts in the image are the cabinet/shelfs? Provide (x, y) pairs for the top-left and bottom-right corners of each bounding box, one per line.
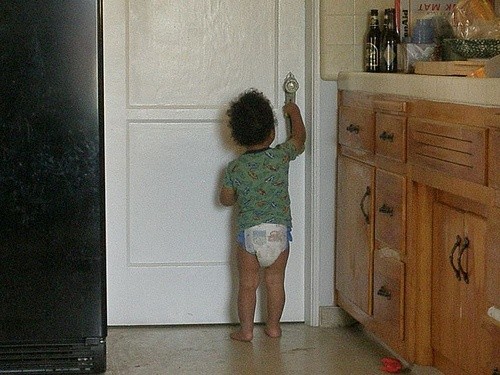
(335, 72), (500, 375)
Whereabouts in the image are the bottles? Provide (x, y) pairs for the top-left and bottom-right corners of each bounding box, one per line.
(364, 8), (381, 72)
(379, 8), (399, 73)
(413, 17), (434, 44)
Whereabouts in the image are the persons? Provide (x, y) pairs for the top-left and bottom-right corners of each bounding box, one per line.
(219, 91), (307, 341)
